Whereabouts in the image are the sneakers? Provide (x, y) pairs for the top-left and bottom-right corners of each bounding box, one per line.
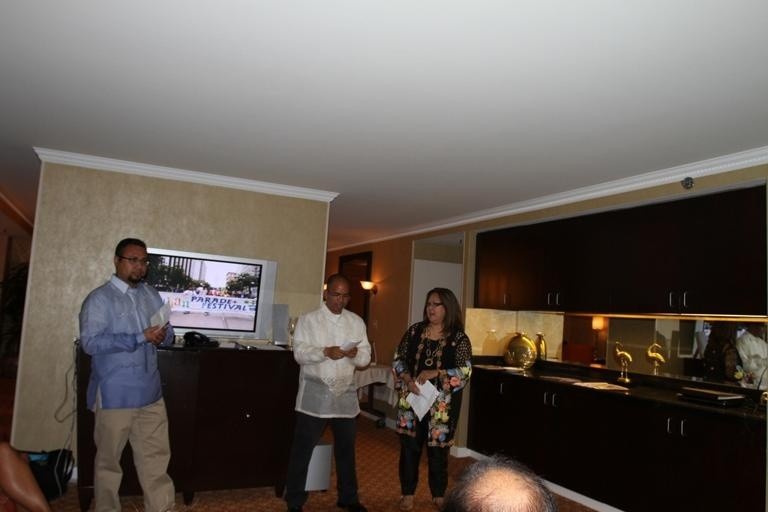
(432, 496), (446, 509)
(400, 495), (415, 510)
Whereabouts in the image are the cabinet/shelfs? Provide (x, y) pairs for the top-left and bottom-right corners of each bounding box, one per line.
(0, 141), (41, 446)
(75, 339), (300, 512)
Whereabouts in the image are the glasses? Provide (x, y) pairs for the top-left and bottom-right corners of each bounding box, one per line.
(115, 255), (150, 266)
(426, 302), (444, 307)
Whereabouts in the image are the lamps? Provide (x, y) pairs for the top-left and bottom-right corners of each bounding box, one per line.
(590, 316), (605, 363)
(360, 280), (377, 294)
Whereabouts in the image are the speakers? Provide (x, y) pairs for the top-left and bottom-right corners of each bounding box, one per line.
(273, 304), (289, 345)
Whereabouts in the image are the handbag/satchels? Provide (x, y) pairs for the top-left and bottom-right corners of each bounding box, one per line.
(14, 447), (76, 500)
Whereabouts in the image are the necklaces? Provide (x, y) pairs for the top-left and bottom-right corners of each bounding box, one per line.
(424, 325), (449, 359)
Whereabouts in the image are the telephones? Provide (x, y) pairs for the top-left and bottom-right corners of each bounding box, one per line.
(184, 332), (218, 347)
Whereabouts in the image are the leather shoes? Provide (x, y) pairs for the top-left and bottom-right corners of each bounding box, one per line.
(335, 498), (369, 512)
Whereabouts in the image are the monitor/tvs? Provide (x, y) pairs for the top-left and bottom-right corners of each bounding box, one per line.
(144, 247), (279, 340)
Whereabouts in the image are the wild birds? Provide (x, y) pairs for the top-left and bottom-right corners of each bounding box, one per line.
(645, 342), (665, 375)
(611, 340), (633, 384)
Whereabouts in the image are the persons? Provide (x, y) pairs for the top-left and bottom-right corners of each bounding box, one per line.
(186, 283), (232, 298)
(284, 274), (372, 512)
(79, 239), (176, 512)
(393, 287), (472, 510)
(0, 442), (52, 512)
(442, 455), (556, 512)
